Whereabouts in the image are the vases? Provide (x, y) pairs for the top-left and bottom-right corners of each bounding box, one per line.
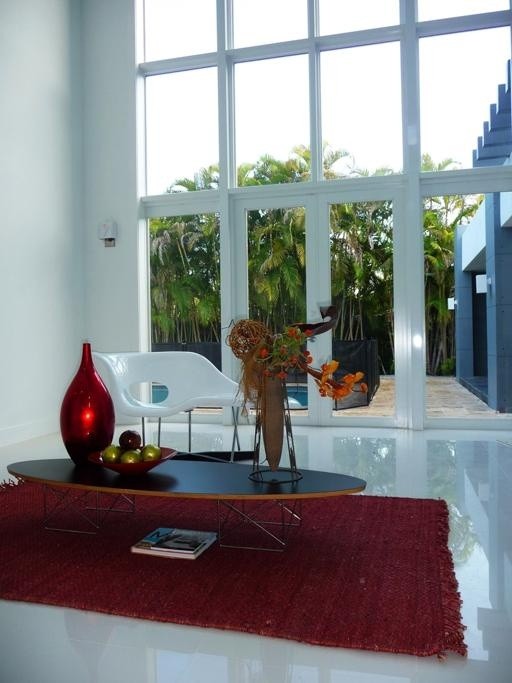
(262, 378), (285, 473)
(60, 343), (116, 467)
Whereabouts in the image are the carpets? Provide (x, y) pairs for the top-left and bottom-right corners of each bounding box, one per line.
(1, 474), (468, 662)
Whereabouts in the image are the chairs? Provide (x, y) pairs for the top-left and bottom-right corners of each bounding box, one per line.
(92, 350), (304, 465)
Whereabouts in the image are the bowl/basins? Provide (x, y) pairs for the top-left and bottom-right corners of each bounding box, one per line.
(85, 442), (177, 474)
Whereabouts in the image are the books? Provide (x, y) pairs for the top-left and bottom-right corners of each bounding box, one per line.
(150, 529), (213, 553)
(131, 527), (218, 560)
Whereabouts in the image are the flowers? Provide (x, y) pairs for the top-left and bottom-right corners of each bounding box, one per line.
(255, 325), (368, 401)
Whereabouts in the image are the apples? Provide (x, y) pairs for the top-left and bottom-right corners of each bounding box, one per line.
(141, 443), (162, 462)
(120, 449), (142, 464)
(102, 445), (120, 464)
(119, 430), (141, 449)
